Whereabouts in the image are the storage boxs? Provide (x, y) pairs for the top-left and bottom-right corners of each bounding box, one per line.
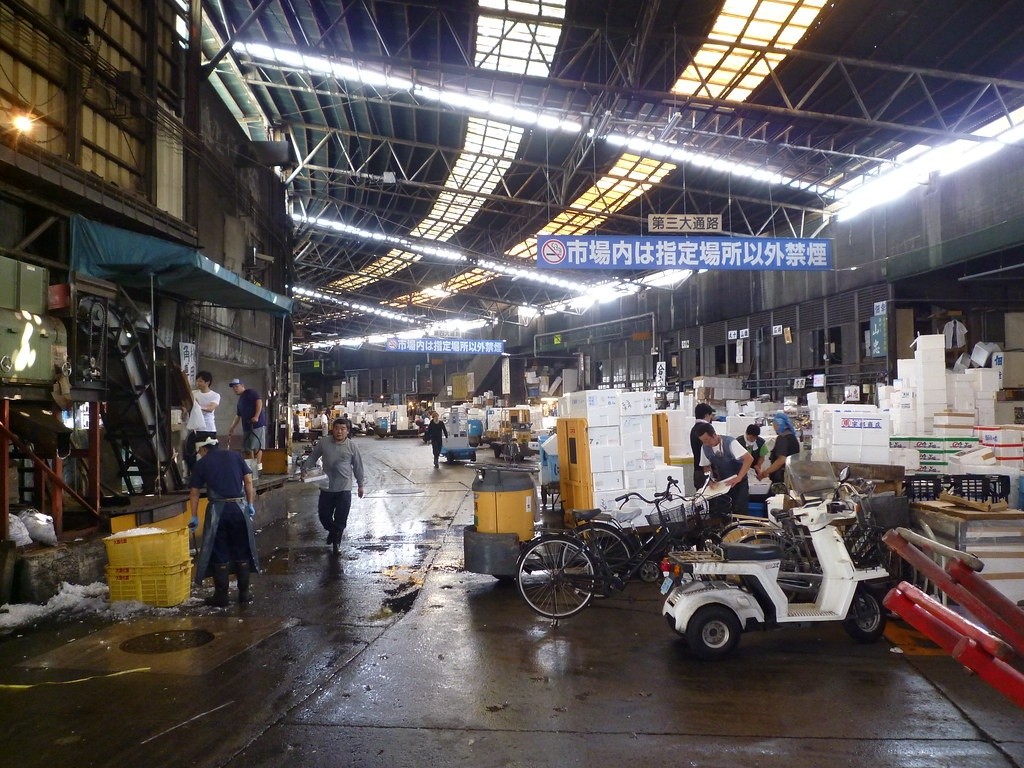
(290, 307), (1024, 603)
(102, 526), (194, 607)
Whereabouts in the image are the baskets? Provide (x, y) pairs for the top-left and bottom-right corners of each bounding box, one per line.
(855, 494), (907, 529)
(645, 504), (686, 540)
(685, 492), (733, 531)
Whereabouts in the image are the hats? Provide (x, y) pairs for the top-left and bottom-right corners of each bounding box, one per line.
(694, 403), (716, 414)
(229, 378), (242, 387)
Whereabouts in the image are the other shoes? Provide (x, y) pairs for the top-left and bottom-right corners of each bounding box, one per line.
(435, 463), (439, 467)
(204, 595), (229, 607)
(333, 544), (342, 554)
(241, 590), (253, 603)
(325, 533), (334, 544)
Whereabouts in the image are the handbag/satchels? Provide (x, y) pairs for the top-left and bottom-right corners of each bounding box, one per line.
(186, 399), (206, 429)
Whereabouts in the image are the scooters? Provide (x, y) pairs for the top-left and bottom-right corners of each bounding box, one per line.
(658, 464), (892, 664)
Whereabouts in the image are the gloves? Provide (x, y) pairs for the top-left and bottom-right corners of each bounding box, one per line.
(189, 516), (200, 532)
(248, 504), (254, 518)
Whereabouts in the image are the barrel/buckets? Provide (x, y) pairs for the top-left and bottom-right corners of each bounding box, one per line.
(472, 469), (537, 542)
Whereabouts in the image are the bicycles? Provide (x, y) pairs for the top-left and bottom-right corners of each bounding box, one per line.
(514, 470), (903, 629)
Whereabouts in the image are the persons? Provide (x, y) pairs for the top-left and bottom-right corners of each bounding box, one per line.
(294, 410), (300, 431)
(754, 414), (800, 517)
(428, 413), (448, 468)
(182, 371), (221, 468)
(301, 419), (364, 544)
(697, 424), (754, 514)
(736, 424), (769, 473)
(690, 403), (713, 490)
(189, 435), (259, 606)
(229, 379), (266, 476)
